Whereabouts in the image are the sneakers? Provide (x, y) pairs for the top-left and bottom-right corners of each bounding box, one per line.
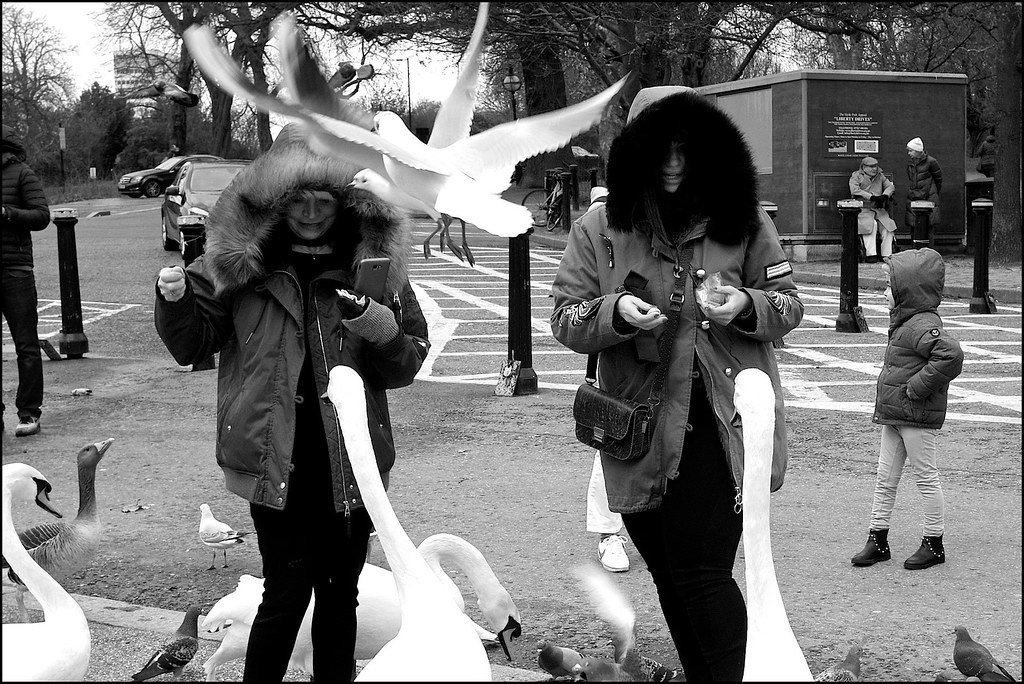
(598, 535), (630, 571)
(15, 416), (40, 437)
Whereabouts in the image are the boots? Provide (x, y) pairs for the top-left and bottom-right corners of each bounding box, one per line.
(851, 529), (891, 564)
(904, 534), (945, 569)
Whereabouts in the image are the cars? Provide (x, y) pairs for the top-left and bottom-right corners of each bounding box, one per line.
(118, 155), (225, 198)
(161, 159), (255, 259)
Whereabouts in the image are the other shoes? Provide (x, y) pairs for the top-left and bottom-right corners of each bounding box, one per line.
(865, 256), (878, 263)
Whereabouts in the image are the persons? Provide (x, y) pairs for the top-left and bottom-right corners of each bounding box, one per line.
(168, 144), (180, 158)
(849, 157), (896, 263)
(852, 247), (964, 570)
(550, 85), (805, 684)
(0, 123), (51, 434)
(154, 121), (431, 681)
(906, 136), (943, 249)
(978, 135), (995, 178)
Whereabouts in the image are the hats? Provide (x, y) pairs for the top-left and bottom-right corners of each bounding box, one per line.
(907, 137), (923, 152)
(862, 157), (878, 165)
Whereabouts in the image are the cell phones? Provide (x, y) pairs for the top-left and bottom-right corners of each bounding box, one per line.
(354, 257), (390, 305)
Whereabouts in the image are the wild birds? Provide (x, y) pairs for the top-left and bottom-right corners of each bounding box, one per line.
(2, 437), (115, 624)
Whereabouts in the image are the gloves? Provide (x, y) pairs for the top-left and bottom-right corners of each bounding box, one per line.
(336, 290), (370, 320)
(870, 195), (888, 209)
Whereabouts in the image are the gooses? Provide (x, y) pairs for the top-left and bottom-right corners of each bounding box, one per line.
(2, 462), (91, 682)
(733, 365), (813, 682)
(203, 534), (522, 682)
(321, 364), (493, 683)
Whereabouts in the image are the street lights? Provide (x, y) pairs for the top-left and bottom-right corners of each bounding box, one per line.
(396, 58), (412, 132)
(502, 65), (522, 185)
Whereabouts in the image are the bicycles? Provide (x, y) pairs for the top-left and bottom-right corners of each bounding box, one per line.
(521, 171), (574, 231)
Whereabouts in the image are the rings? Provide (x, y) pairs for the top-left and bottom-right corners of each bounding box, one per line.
(170, 290), (176, 296)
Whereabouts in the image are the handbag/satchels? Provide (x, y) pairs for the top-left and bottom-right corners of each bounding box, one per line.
(573, 384), (651, 461)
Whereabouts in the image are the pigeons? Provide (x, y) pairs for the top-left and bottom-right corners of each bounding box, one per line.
(534, 632), (683, 682)
(120, 79), (198, 107)
(130, 605), (204, 682)
(181, 2), (633, 268)
(198, 503), (256, 571)
(813, 645), (869, 682)
(935, 626), (1016, 682)
